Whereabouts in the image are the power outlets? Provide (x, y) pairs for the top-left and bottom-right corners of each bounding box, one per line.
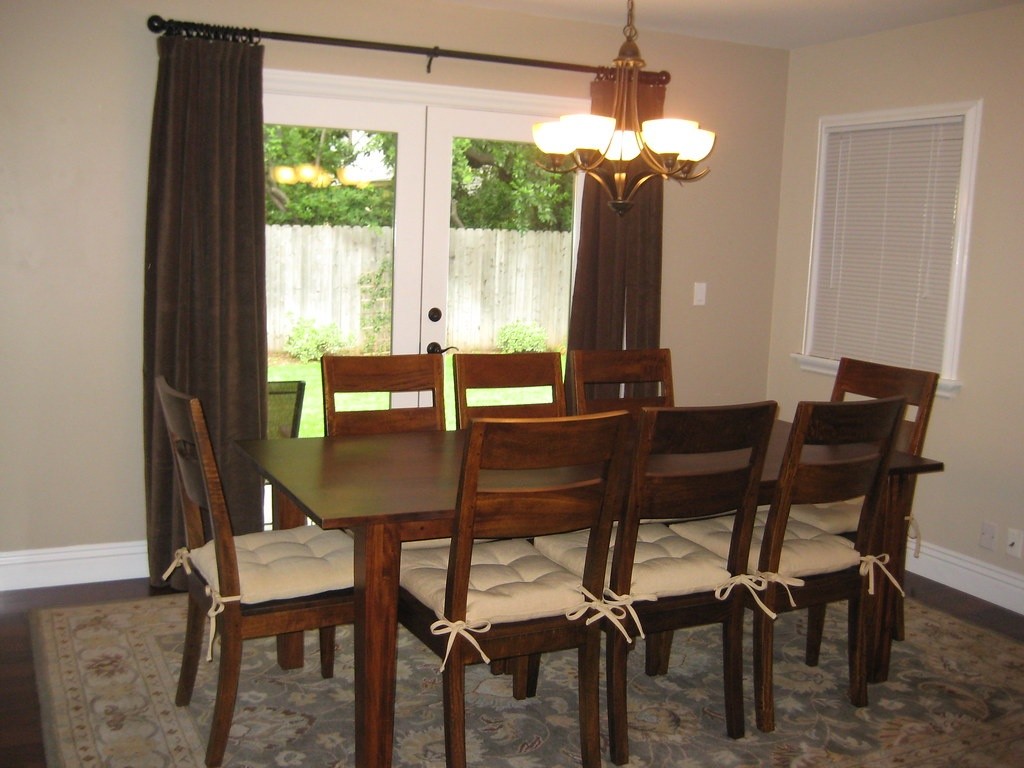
(978, 519), (1000, 552)
(1006, 527), (1024, 559)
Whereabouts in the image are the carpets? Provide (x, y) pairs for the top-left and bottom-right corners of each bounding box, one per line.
(30, 584), (1024, 768)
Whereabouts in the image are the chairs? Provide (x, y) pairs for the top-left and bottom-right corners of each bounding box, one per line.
(566, 349), (675, 417)
(524, 400), (776, 767)
(396, 405), (642, 768)
(659, 394), (907, 735)
(320, 353), (447, 438)
(153, 374), (377, 767)
(770, 357), (939, 684)
(453, 352), (567, 430)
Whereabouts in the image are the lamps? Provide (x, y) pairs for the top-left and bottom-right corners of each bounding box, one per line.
(532, 0), (716, 216)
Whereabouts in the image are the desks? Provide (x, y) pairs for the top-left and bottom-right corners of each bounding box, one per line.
(232, 417), (945, 767)
